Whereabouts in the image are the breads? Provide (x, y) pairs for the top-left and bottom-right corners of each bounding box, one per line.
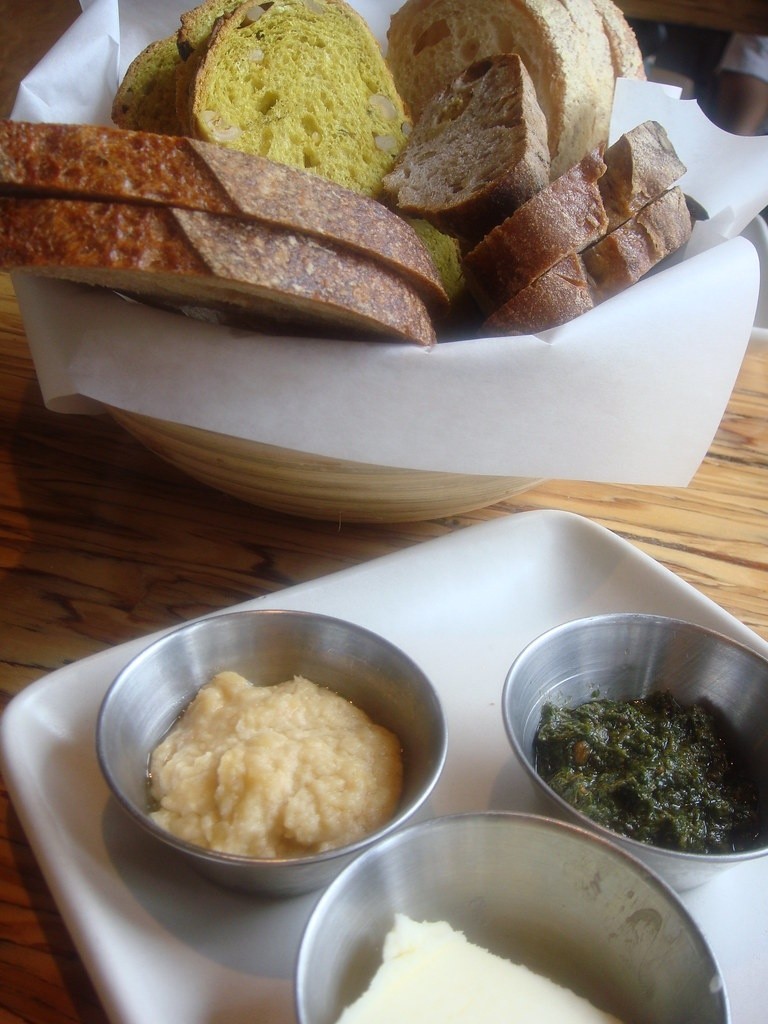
(0, 0), (698, 347)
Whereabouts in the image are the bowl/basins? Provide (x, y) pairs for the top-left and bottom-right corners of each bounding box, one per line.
(297, 812), (729, 1024)
(503, 615), (768, 860)
(97, 611), (446, 896)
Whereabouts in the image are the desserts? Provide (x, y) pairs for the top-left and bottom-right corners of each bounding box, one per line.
(333, 913), (632, 1024)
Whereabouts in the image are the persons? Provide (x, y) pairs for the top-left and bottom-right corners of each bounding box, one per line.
(714, 33), (768, 136)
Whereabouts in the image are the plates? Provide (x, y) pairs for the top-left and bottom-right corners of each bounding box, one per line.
(0, 509), (768, 1023)
